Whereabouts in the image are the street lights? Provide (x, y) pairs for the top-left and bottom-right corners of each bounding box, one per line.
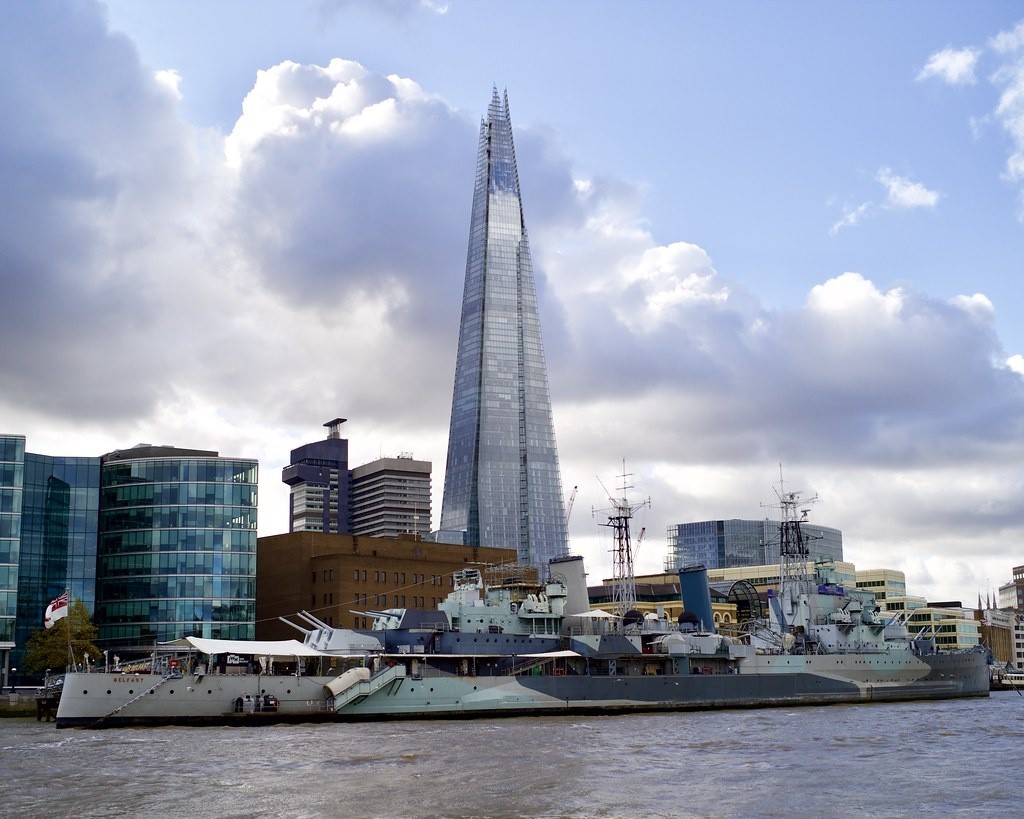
(10, 667), (18, 694)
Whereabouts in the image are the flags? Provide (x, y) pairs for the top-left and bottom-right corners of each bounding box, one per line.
(51, 592), (68, 621)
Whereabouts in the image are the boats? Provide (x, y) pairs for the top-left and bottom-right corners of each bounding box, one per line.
(54, 562), (996, 785)
